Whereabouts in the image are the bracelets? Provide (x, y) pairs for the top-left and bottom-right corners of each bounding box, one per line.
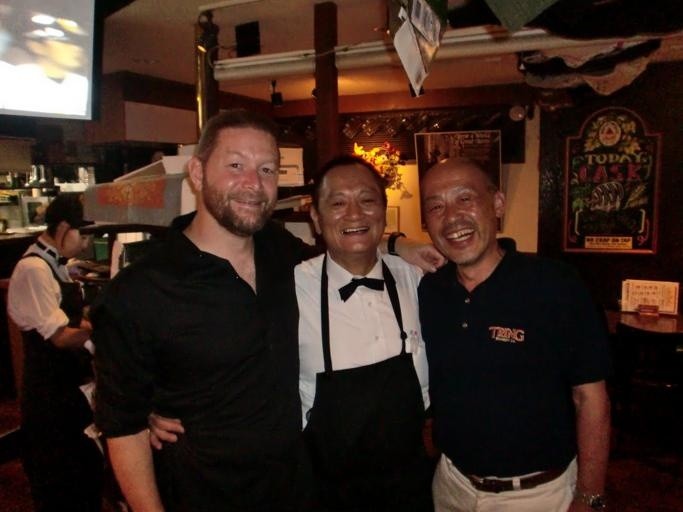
(388, 232), (406, 254)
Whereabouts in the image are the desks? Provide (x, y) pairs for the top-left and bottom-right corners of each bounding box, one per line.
(609, 308), (681, 353)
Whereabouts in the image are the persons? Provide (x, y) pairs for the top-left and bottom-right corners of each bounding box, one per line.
(418, 157), (614, 512)
(147, 154), (430, 511)
(87, 110), (449, 511)
(7, 194), (105, 512)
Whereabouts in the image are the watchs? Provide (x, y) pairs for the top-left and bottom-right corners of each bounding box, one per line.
(573, 492), (609, 512)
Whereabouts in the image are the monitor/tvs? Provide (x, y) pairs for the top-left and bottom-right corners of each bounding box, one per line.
(0, 0), (103, 123)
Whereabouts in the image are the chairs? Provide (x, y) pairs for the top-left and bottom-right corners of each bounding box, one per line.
(608, 322), (682, 464)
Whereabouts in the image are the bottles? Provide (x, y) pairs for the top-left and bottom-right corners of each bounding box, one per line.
(25, 164), (48, 188)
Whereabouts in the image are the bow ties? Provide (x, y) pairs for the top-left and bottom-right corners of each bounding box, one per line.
(338, 276), (385, 302)
(57, 256), (68, 266)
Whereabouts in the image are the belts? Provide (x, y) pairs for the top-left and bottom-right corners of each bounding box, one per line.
(463, 456), (574, 494)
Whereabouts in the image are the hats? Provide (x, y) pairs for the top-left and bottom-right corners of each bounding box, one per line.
(45, 192), (95, 233)
